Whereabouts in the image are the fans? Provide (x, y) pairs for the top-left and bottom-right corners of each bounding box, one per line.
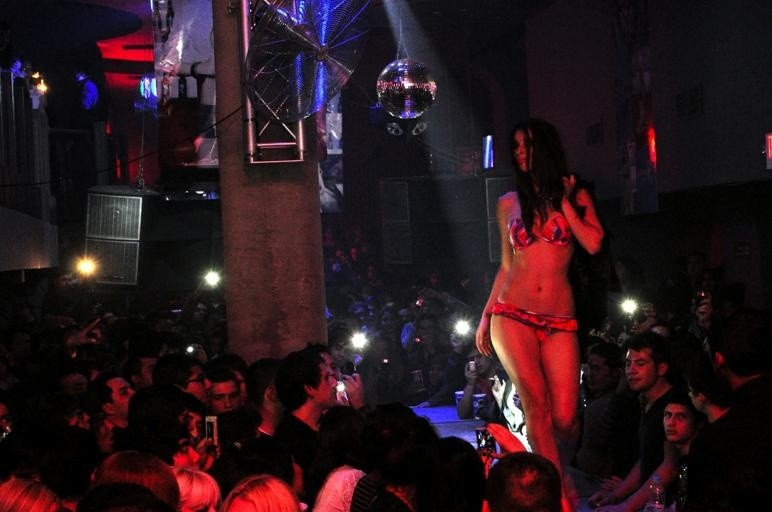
(227, 0), (375, 154)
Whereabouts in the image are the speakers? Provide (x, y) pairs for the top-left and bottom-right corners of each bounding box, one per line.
(84, 193), (143, 242)
(485, 175), (516, 220)
(84, 238), (139, 285)
(487, 219), (503, 263)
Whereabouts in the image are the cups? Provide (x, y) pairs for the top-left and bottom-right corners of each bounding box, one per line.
(472, 393), (488, 420)
(646, 480), (666, 512)
(455, 391), (465, 411)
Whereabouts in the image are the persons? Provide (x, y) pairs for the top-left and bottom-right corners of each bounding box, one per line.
(1, 117), (772, 511)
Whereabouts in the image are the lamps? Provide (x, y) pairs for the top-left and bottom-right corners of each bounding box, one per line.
(376, 0), (437, 119)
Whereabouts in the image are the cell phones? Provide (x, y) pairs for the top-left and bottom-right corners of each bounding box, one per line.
(205, 414), (218, 446)
(468, 360), (476, 370)
(475, 426), (497, 457)
